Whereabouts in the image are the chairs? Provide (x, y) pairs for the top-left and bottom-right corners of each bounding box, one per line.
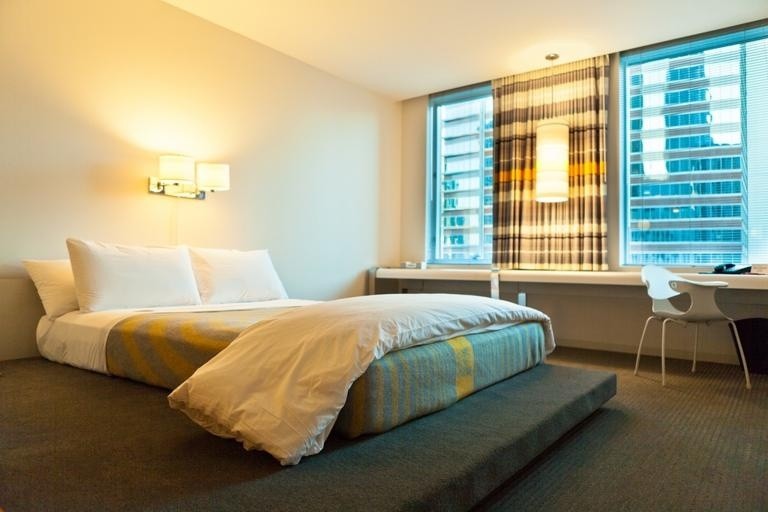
(633, 264), (752, 390)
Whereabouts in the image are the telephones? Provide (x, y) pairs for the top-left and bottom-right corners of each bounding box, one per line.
(714, 264), (752, 274)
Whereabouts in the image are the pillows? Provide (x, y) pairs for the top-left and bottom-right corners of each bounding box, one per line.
(20, 237), (290, 321)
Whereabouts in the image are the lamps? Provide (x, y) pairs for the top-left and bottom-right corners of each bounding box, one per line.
(536, 52), (570, 202)
(148, 154), (231, 200)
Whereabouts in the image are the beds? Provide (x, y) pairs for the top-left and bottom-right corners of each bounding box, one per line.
(0, 264), (546, 440)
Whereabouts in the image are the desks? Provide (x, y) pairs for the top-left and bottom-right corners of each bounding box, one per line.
(368, 266), (768, 368)
